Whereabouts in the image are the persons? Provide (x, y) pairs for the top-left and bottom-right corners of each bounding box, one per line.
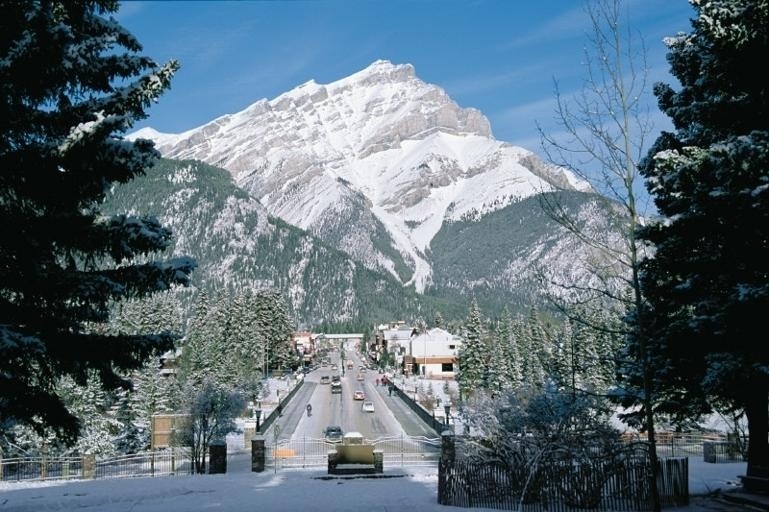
(277, 404), (282, 414)
(307, 404), (312, 416)
(376, 379), (379, 386)
(388, 384), (392, 396)
(383, 375), (388, 386)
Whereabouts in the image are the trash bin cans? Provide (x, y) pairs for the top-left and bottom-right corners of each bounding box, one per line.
(209, 440), (227, 474)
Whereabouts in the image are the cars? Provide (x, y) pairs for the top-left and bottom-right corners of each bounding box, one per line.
(354, 390), (365, 400)
(320, 356), (369, 393)
(361, 401), (375, 412)
(322, 425), (343, 443)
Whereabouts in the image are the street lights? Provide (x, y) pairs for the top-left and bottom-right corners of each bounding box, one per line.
(443, 396), (453, 430)
(254, 407), (262, 433)
